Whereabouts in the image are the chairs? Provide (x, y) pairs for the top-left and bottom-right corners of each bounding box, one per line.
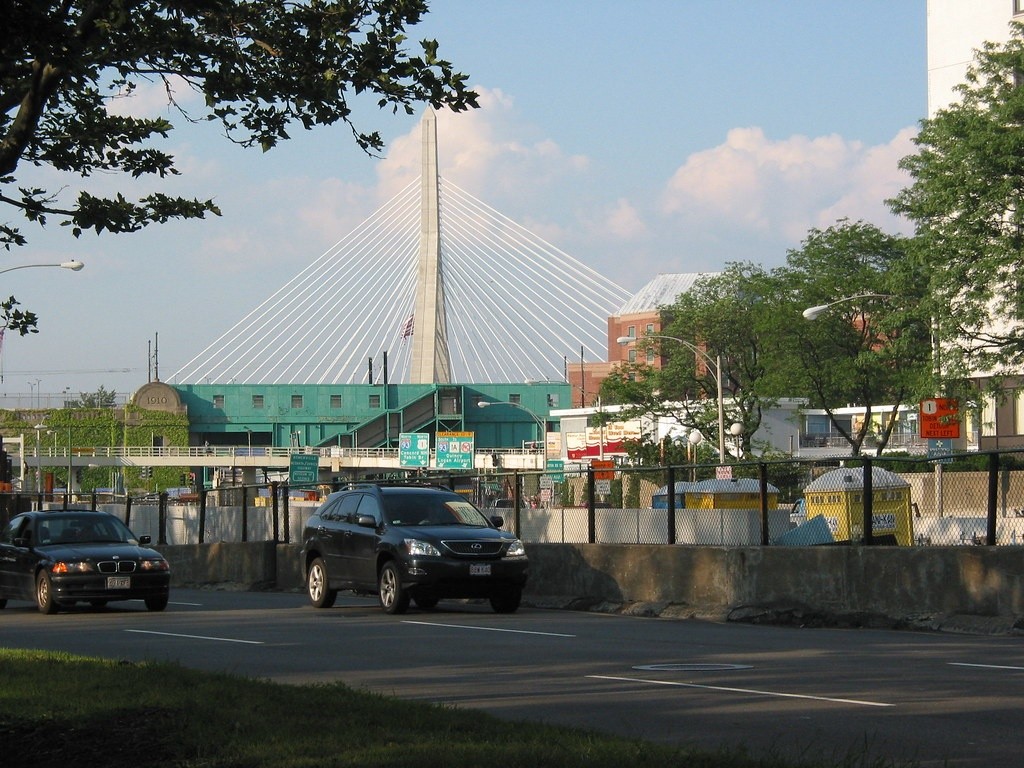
(80, 526), (99, 542)
(39, 527), (50, 541)
(61, 529), (76, 543)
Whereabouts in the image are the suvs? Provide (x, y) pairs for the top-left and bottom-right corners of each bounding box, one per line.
(299, 483), (530, 615)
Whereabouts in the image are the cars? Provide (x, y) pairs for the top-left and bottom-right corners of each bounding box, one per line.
(0, 508), (171, 617)
(790, 499), (807, 526)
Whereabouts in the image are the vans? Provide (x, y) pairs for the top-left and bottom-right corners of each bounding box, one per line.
(490, 499), (530, 510)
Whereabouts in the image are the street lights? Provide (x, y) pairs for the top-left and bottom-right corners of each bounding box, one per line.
(803, 294), (944, 518)
(35, 423), (73, 503)
(525, 379), (605, 461)
(616, 335), (725, 464)
(731, 422), (743, 462)
(689, 432), (702, 481)
(477, 402), (548, 471)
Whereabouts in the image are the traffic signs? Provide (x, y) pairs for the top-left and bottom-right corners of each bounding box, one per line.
(436, 431), (474, 469)
(399, 433), (429, 466)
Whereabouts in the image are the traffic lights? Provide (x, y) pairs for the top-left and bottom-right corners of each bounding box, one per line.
(141, 467), (146, 478)
(190, 474), (195, 486)
(180, 474), (185, 486)
(148, 466), (153, 479)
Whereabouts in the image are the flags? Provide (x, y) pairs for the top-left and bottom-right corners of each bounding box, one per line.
(401, 315), (414, 343)
